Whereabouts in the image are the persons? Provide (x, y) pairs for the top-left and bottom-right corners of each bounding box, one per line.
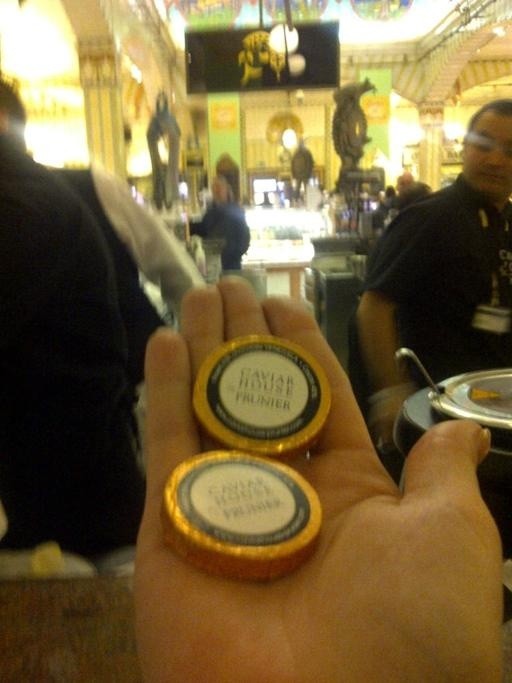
(130, 272), (504, 683)
(351, 95), (511, 622)
(0, 80), (202, 456)
(371, 185), (400, 231)
(0, 129), (147, 569)
(387, 170), (431, 212)
(190, 173), (251, 270)
